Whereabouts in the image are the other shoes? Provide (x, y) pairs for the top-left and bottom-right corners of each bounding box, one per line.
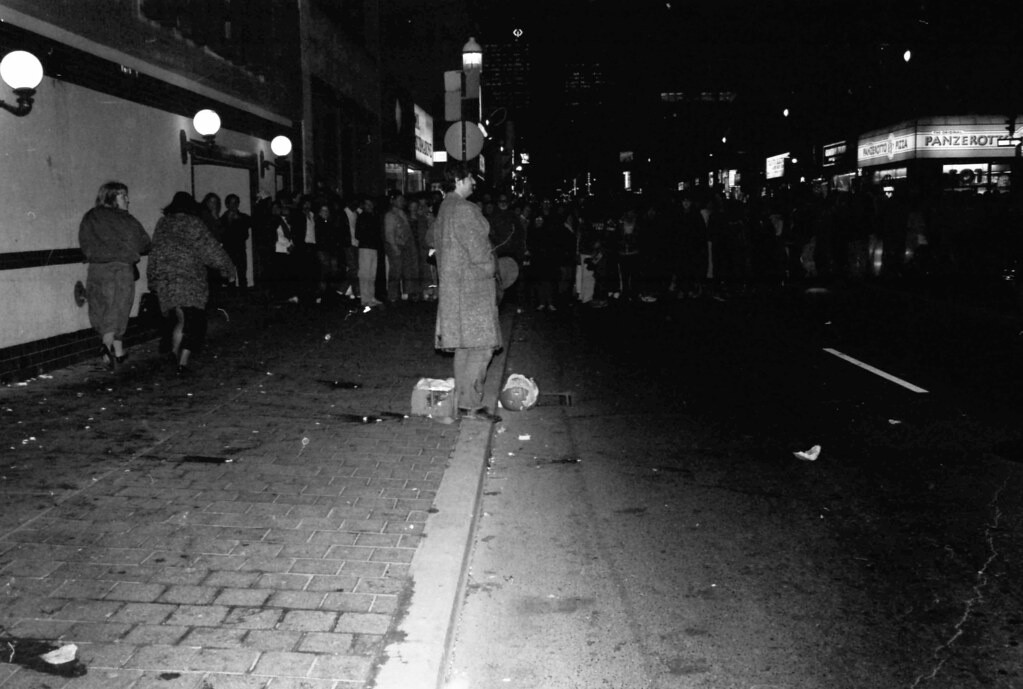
(208, 280), (684, 334)
(174, 366), (192, 376)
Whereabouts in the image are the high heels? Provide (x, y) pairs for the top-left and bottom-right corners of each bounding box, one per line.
(99, 344), (115, 370)
(115, 351), (130, 364)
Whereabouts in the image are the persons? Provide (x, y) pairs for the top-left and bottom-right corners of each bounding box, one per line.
(146, 191), (238, 375)
(219, 194), (252, 302)
(480, 166), (1022, 318)
(256, 188), (440, 311)
(80, 181), (152, 368)
(431, 163), (504, 422)
(198, 193), (228, 312)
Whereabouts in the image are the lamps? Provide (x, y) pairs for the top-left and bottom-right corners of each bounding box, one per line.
(179, 109), (222, 165)
(0, 50), (43, 116)
(260, 135), (292, 179)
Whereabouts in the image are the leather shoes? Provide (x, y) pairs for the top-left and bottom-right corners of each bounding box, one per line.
(458, 408), (468, 416)
(469, 409), (502, 422)
